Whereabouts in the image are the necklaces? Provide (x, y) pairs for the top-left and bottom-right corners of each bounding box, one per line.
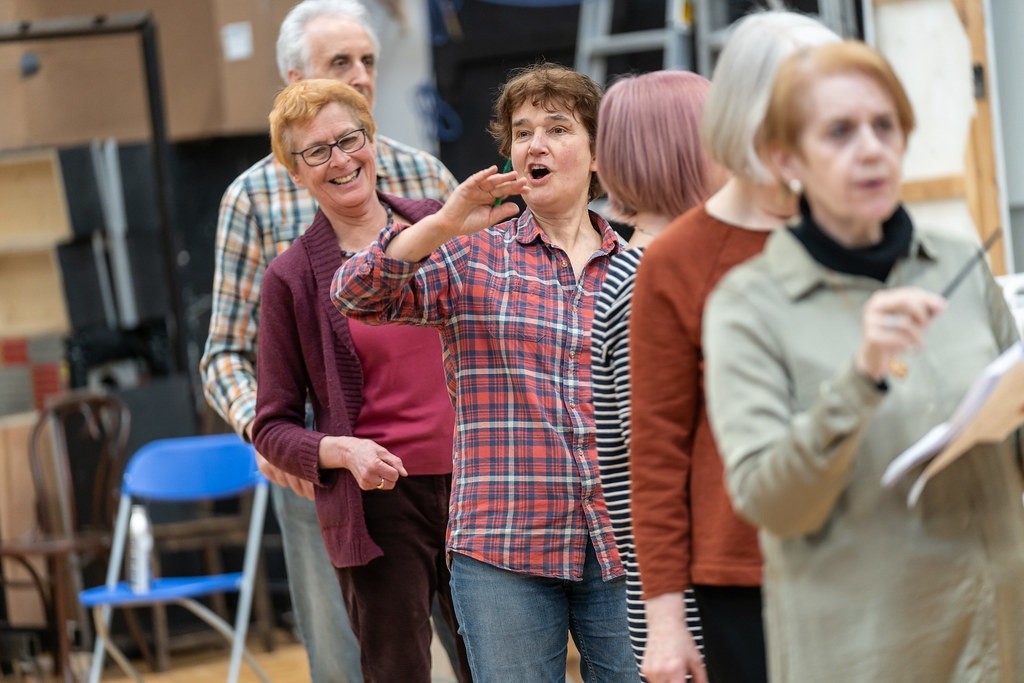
(341, 202), (394, 258)
(633, 226), (657, 238)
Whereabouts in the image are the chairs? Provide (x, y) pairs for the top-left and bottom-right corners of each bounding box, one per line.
(0, 394), (299, 683)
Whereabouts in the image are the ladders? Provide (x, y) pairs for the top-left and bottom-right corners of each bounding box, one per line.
(567, 0), (859, 94)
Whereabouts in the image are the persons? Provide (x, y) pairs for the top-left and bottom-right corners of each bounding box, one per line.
(199, 0), (1024, 683)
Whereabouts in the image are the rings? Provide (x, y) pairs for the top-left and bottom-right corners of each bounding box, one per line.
(377, 478), (385, 489)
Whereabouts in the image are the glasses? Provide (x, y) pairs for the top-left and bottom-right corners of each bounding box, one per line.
(291, 128), (366, 166)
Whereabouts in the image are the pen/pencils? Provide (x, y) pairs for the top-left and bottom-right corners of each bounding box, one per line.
(936, 226), (1005, 299)
(494, 157), (513, 205)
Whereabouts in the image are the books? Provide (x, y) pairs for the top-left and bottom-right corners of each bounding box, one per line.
(882, 341), (1024, 507)
